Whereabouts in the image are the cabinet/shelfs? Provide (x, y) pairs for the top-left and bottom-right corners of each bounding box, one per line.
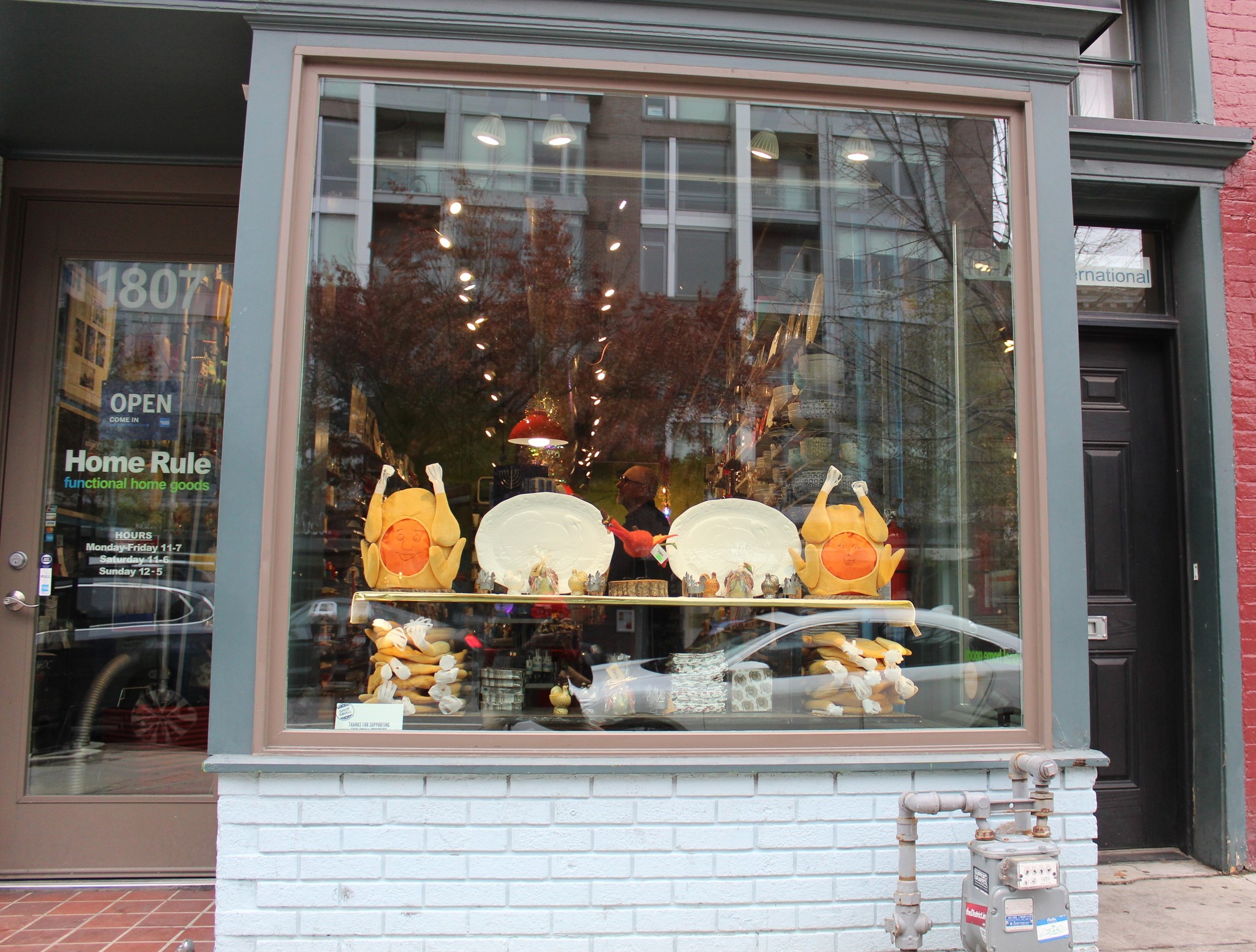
(350, 475), (924, 730)
(698, 191), (884, 678)
(26, 261), (423, 749)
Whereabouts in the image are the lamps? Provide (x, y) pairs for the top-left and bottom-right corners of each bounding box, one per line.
(491, 456), (506, 467)
(472, 111), (506, 146)
(542, 113), (577, 145)
(569, 197), (628, 490)
(842, 129), (875, 161)
(750, 126), (779, 160)
(437, 198), (508, 438)
(507, 310), (568, 448)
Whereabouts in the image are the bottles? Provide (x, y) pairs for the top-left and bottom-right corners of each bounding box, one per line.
(526, 649), (553, 675)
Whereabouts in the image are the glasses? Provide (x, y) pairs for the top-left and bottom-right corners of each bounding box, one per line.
(619, 476), (645, 487)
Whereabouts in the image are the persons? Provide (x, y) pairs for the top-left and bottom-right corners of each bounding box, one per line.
(604, 466), (669, 622)
(508, 602), (592, 711)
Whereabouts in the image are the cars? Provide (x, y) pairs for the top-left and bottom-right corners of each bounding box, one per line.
(289, 595), (486, 696)
(563, 608), (1020, 730)
(28, 576), (216, 754)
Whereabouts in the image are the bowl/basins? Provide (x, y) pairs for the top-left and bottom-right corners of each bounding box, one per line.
(753, 352), (860, 504)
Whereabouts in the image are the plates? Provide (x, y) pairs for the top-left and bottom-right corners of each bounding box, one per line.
(757, 273), (823, 367)
(474, 492), (615, 594)
(665, 497), (802, 597)
